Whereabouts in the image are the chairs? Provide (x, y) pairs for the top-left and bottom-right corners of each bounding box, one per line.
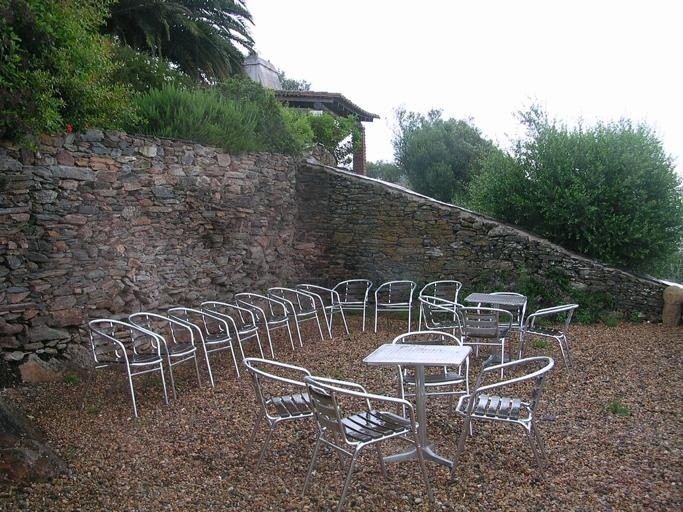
(456, 307), (514, 379)
(242, 357), (345, 476)
(77, 283), (350, 418)
(328, 278), (374, 337)
(517, 303), (580, 371)
(418, 280), (463, 342)
(451, 355), (555, 479)
(374, 280), (417, 334)
(418, 295), (465, 346)
(391, 329), (473, 438)
(475, 291), (528, 360)
(300, 375), (435, 512)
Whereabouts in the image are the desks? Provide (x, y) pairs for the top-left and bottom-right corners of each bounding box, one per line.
(361, 343), (474, 469)
(464, 292), (528, 366)
